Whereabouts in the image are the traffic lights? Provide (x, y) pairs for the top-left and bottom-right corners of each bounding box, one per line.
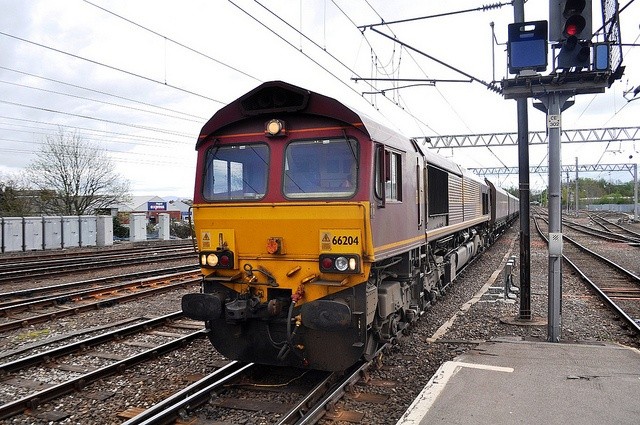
(558, 37), (590, 70)
(559, 0), (587, 38)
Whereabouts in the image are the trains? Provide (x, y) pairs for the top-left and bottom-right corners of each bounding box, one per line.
(181, 81), (520, 375)
